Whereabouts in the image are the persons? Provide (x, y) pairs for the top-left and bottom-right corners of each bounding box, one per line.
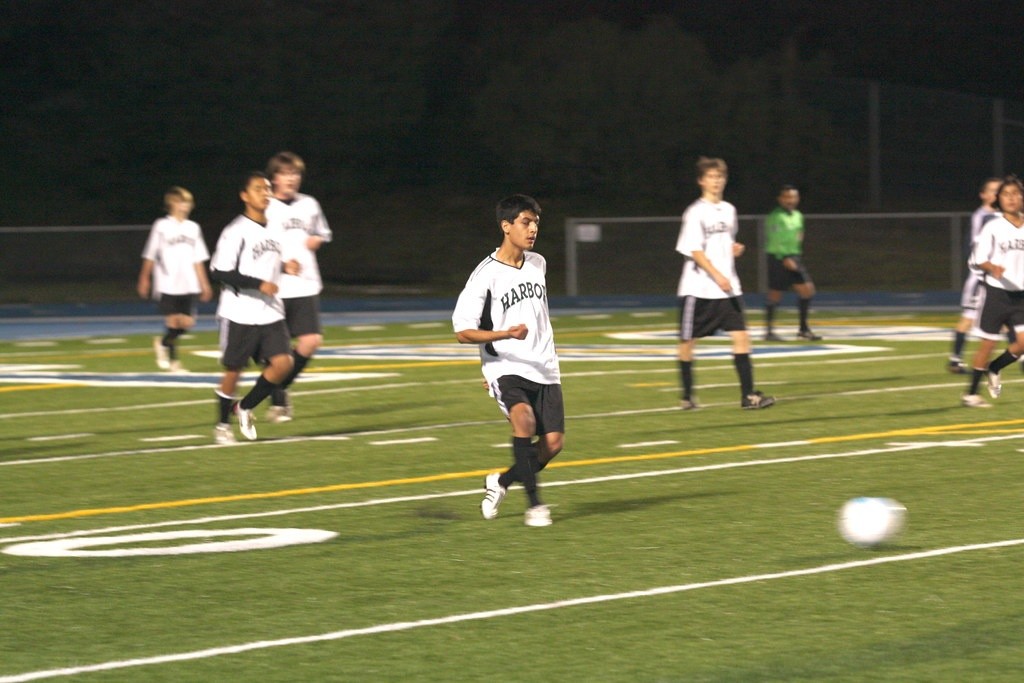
(676, 159), (777, 410)
(209, 172), (303, 445)
(764, 188), (822, 340)
(452, 195), (565, 528)
(255, 151), (333, 423)
(138, 188), (212, 369)
(947, 178), (1024, 409)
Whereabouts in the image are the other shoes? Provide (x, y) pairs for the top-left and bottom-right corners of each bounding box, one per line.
(985, 369), (1002, 398)
(154, 337), (170, 370)
(763, 330), (787, 345)
(962, 393), (994, 409)
(265, 405), (292, 422)
(169, 355), (188, 371)
(797, 330), (821, 340)
(947, 357), (970, 371)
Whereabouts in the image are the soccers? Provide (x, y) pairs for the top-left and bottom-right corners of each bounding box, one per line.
(842, 496), (889, 544)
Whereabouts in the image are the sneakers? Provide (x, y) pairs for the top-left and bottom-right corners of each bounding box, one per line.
(232, 400), (258, 440)
(214, 424), (233, 446)
(740, 392), (776, 408)
(527, 505), (553, 527)
(681, 394), (701, 413)
(479, 472), (506, 520)
